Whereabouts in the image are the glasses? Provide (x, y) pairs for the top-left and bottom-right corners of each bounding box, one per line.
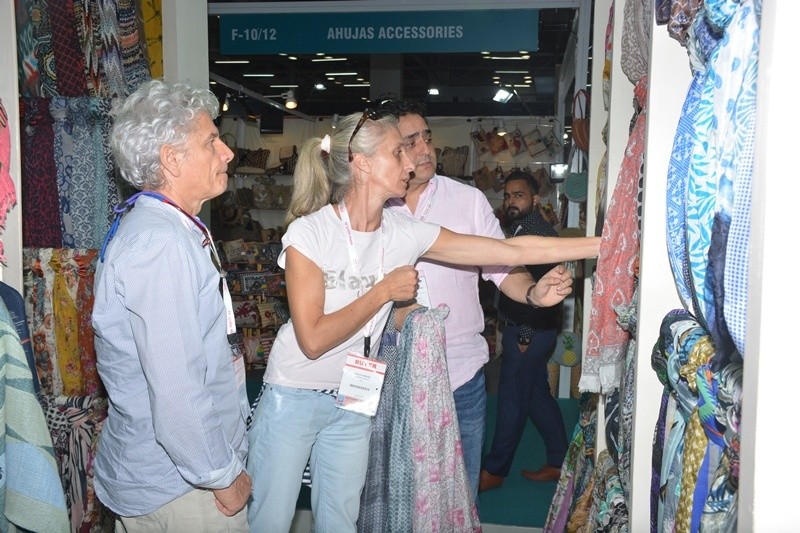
(348, 104), (390, 162)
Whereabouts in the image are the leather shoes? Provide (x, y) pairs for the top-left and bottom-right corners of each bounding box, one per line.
(479, 467), (502, 491)
(522, 464), (561, 481)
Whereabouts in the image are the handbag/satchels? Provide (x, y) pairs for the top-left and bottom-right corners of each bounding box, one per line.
(470, 122), (555, 195)
(559, 228), (586, 238)
(564, 143), (588, 202)
(571, 89), (591, 152)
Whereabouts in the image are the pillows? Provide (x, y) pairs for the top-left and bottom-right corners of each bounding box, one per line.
(214, 238), (290, 363)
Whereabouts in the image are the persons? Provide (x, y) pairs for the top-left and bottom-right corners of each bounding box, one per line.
(246, 102), (602, 533)
(91, 80), (254, 533)
(477, 171), (567, 491)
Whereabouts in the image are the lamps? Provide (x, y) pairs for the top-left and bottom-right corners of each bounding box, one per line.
(492, 86), (514, 104)
(281, 90), (297, 109)
(223, 98), (229, 111)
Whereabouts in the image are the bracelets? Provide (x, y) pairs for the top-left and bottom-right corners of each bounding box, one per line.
(525, 285), (540, 309)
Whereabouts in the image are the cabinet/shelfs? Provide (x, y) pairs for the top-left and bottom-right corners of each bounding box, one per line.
(225, 260), (288, 373)
